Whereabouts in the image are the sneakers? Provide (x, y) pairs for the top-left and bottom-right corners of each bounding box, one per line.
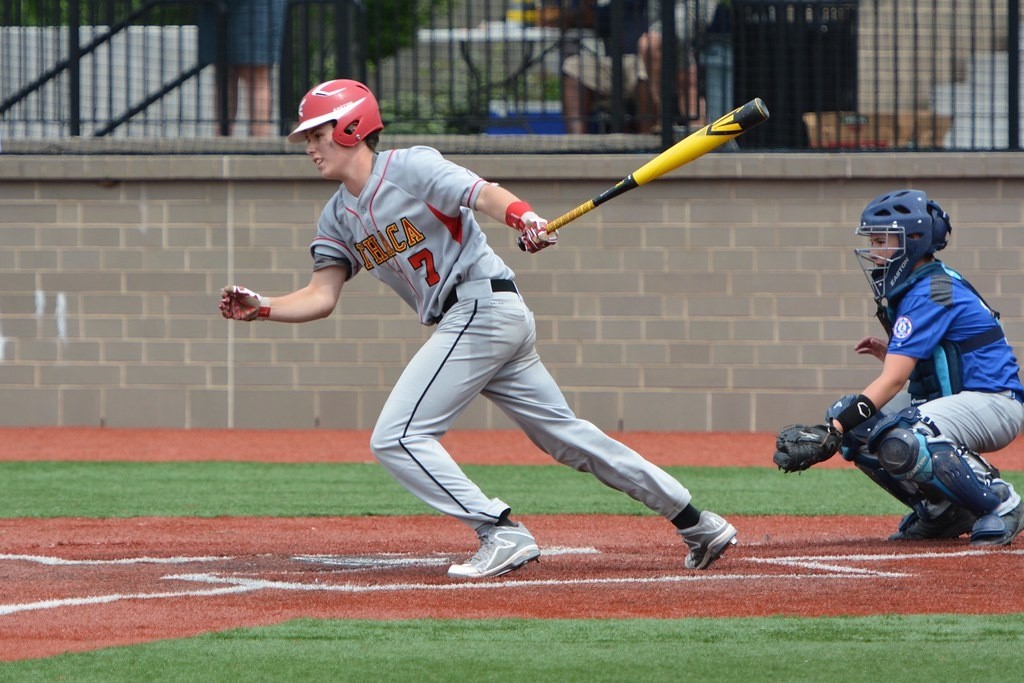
(887, 478), (1024, 547)
(671, 510), (738, 570)
(447, 521), (543, 579)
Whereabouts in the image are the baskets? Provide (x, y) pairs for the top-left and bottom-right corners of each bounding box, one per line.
(799, 110), (954, 151)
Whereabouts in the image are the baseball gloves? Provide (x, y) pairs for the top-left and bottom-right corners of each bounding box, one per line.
(772, 426), (844, 474)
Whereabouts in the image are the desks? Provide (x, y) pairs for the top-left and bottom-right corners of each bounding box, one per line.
(419, 26), (595, 136)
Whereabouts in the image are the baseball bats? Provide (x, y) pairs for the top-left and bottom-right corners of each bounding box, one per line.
(543, 97), (770, 234)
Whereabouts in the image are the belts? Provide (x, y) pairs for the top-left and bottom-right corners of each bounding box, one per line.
(434, 278), (529, 325)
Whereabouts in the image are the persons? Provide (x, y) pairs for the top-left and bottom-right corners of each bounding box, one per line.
(197, 0), (717, 137)
(774, 187), (1024, 547)
(219, 80), (739, 579)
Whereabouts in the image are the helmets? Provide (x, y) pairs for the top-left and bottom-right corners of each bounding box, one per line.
(852, 188), (953, 307)
(286, 78), (385, 148)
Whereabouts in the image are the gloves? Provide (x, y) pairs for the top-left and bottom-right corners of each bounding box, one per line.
(218, 284), (272, 322)
(505, 200), (560, 254)
